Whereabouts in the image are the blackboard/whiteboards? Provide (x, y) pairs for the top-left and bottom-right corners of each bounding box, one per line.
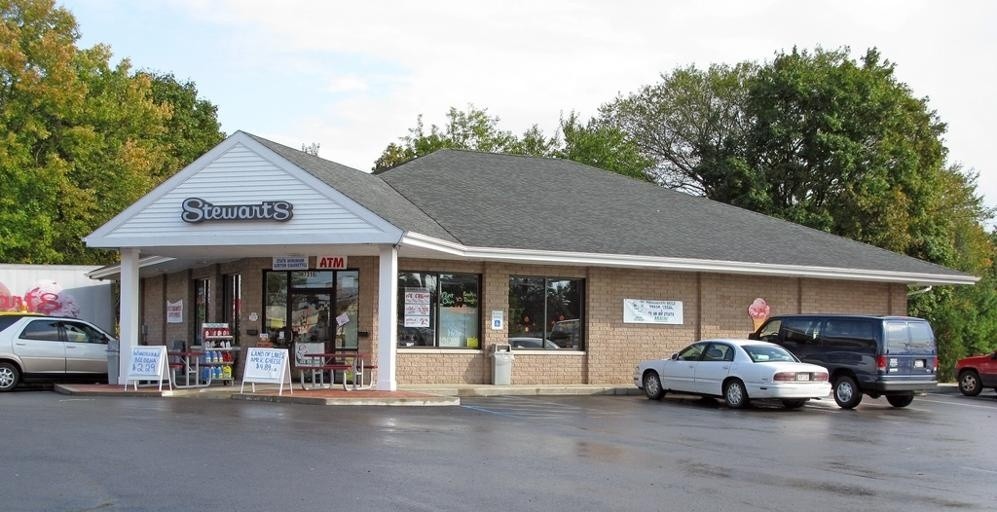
(243, 348), (288, 384)
(126, 346), (166, 381)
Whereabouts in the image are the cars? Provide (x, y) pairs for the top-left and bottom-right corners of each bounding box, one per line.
(955, 349), (997, 395)
(0, 316), (118, 391)
(633, 338), (832, 407)
(508, 338), (560, 350)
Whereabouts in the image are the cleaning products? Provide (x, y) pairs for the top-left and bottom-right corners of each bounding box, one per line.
(203, 330), (232, 380)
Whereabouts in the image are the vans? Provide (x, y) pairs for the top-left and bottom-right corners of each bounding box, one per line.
(552, 319), (580, 348)
(749, 313), (939, 410)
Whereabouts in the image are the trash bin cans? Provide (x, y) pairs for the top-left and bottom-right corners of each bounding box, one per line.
(490, 344), (514, 385)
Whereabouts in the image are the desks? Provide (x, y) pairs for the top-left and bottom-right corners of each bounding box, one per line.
(304, 352), (373, 391)
(168, 352), (207, 389)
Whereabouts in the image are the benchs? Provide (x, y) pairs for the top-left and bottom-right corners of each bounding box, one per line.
(167, 362), (218, 389)
(295, 362), (379, 391)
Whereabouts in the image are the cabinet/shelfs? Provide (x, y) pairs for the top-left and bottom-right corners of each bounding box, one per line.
(200, 322), (234, 386)
